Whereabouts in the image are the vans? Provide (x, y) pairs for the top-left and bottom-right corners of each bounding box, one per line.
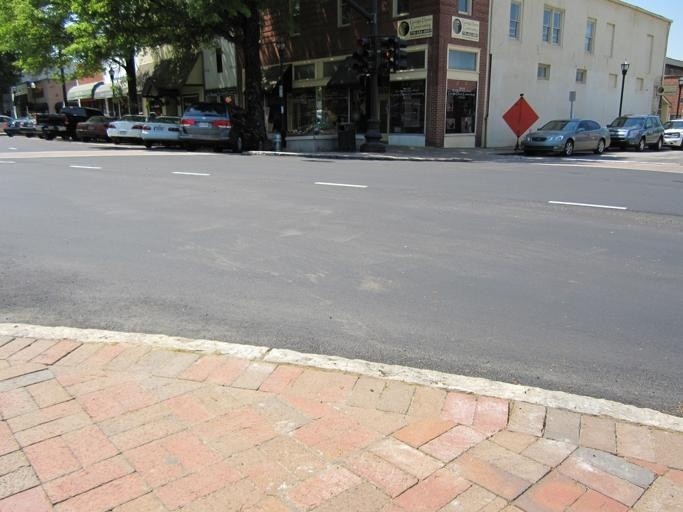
(178, 101), (247, 152)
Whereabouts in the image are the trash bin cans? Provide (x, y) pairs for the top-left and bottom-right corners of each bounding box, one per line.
(336, 121), (356, 152)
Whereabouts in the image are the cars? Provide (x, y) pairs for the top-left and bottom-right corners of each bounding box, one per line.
(606, 114), (665, 152)
(0, 104), (183, 150)
(662, 119), (683, 151)
(523, 118), (611, 156)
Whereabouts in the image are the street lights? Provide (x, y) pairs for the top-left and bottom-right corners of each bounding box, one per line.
(276, 39), (286, 148)
(109, 67), (116, 118)
(618, 60), (630, 116)
(676, 77), (683, 115)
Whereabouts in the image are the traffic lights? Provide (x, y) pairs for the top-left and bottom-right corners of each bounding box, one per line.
(346, 35), (408, 77)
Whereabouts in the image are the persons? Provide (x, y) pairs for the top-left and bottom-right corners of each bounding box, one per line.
(326, 109), (339, 126)
(268, 93), (282, 131)
